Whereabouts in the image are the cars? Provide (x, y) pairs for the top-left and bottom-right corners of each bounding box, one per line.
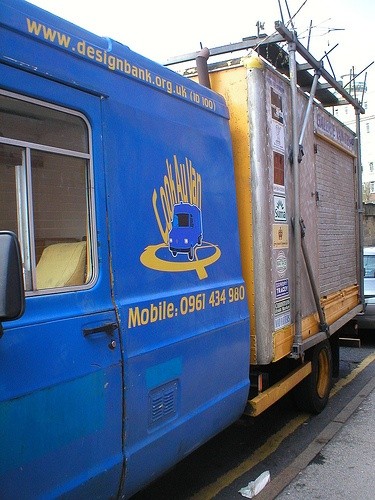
(352, 247), (375, 335)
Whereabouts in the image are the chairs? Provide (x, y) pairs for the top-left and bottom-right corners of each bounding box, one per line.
(35, 241), (87, 289)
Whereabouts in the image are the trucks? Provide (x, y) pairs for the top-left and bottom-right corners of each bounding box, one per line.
(0, 0), (374, 500)
